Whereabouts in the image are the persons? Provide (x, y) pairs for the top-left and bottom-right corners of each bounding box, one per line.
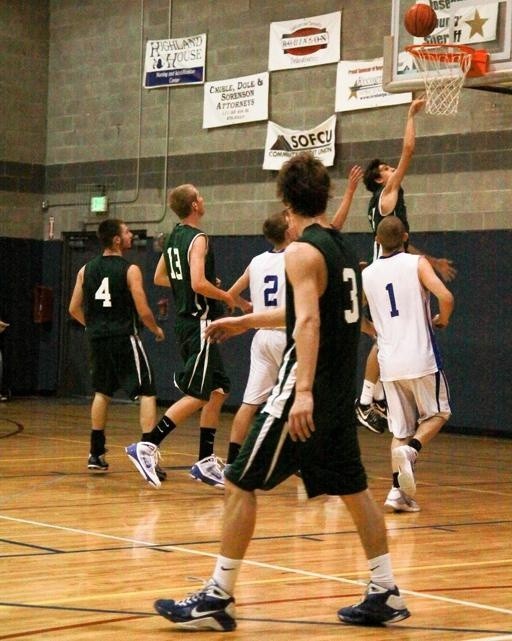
(67, 219), (168, 480)
(359, 214), (455, 514)
(350, 91), (459, 434)
(125, 184), (237, 489)
(220, 164), (365, 480)
(153, 151), (411, 632)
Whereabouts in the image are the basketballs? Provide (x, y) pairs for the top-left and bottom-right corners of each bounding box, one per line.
(404, 4), (436, 36)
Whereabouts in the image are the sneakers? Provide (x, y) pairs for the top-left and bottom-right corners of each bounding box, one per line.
(384, 445), (420, 511)
(154, 577), (237, 631)
(124, 441), (166, 490)
(190, 454), (225, 489)
(338, 581), (411, 624)
(87, 449), (108, 470)
(354, 397), (388, 434)
